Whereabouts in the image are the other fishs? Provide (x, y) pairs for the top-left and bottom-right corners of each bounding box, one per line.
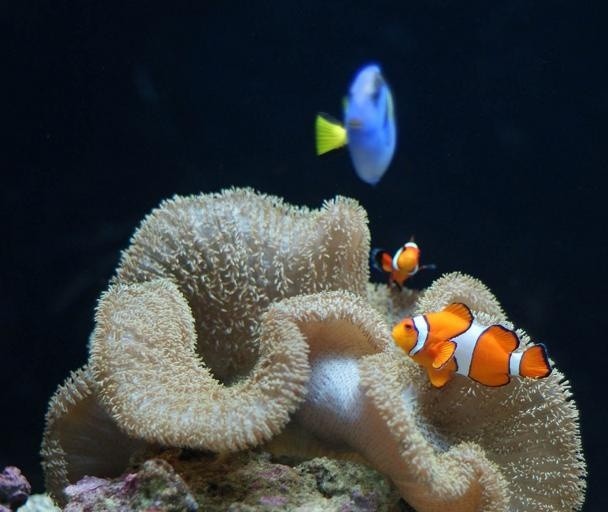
(370, 238), (425, 287)
(389, 300), (555, 390)
(312, 62), (404, 186)
